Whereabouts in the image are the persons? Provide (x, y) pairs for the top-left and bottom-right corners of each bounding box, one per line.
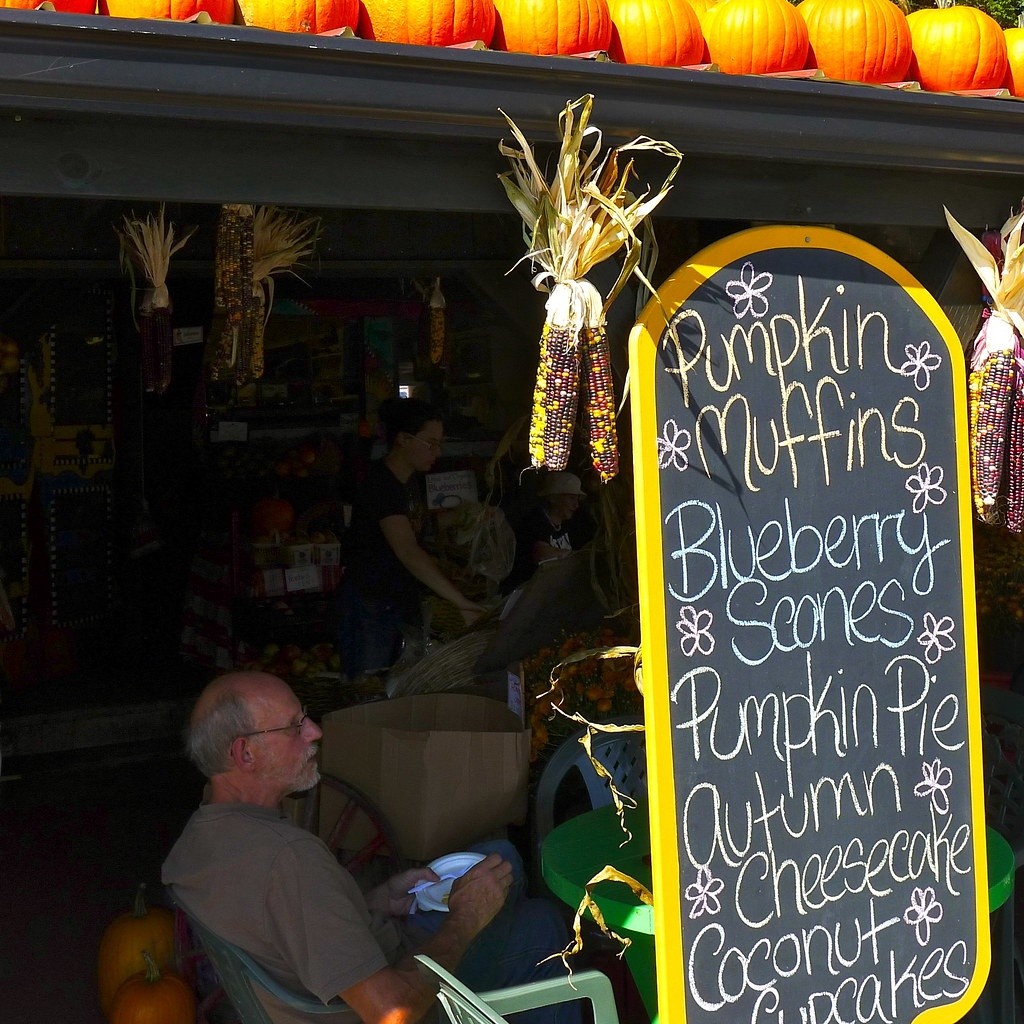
(331, 396), (492, 676)
(512, 469), (592, 580)
(160, 669), (584, 1023)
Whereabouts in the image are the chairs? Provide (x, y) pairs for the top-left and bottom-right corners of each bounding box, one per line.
(982, 683), (1024, 884)
(159, 883), (621, 1024)
(531, 711), (647, 901)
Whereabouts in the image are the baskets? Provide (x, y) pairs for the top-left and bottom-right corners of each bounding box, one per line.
(274, 661), (342, 723)
(418, 577), (501, 639)
(343, 671), (397, 703)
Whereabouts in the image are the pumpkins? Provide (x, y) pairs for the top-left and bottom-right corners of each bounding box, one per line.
(1, 0), (1023, 100)
(100, 879), (197, 1024)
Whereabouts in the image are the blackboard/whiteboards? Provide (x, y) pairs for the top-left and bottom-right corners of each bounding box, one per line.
(623, 226), (996, 1024)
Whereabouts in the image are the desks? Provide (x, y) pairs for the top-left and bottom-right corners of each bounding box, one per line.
(537, 799), (1016, 1024)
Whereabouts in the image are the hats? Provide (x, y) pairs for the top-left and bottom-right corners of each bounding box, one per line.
(535, 472), (591, 500)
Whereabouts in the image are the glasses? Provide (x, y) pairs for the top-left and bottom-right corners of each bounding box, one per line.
(236, 701), (310, 742)
(401, 432), (446, 453)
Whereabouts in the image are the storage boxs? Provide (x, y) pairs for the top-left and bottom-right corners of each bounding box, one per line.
(318, 693), (534, 879)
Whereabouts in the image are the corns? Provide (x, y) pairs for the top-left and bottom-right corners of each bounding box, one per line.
(943, 309), (1024, 536)
(421, 291), (448, 363)
(525, 283), (624, 482)
(135, 203), (268, 392)
